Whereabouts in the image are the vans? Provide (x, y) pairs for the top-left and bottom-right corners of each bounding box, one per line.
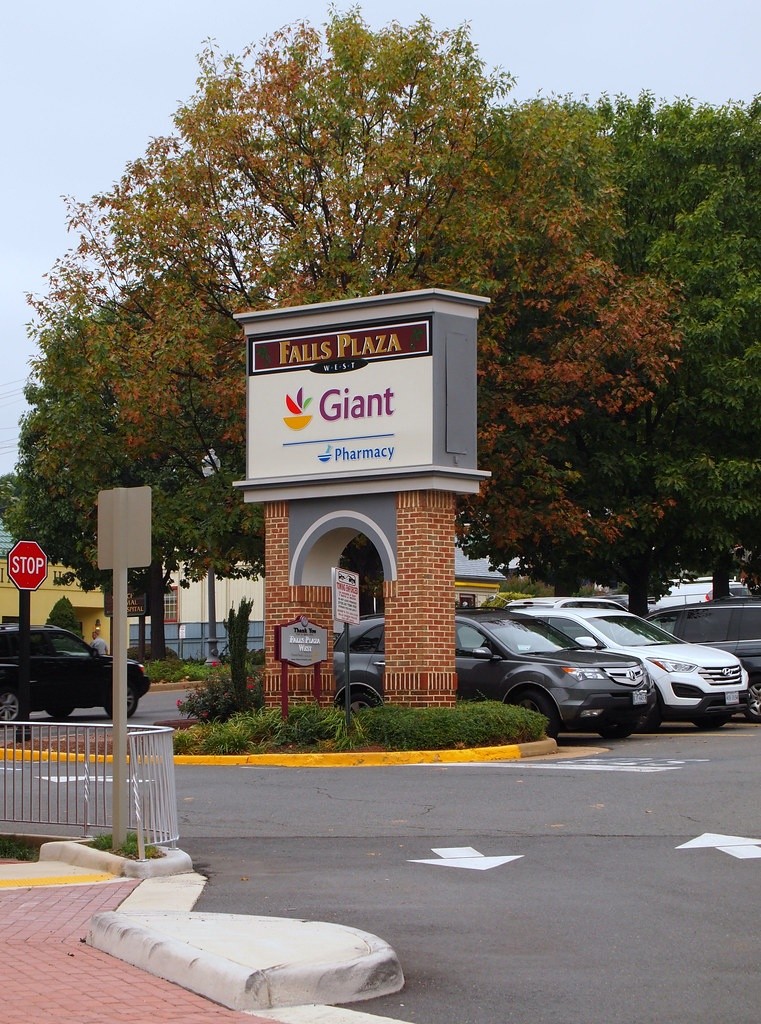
(647, 576), (751, 613)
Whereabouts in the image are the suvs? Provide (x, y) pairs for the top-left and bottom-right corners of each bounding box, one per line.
(617, 595), (761, 725)
(331, 605), (657, 742)
(458, 605), (751, 735)
(502, 596), (629, 640)
(0, 621), (151, 727)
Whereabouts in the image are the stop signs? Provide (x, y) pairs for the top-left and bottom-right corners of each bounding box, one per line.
(6, 541), (49, 591)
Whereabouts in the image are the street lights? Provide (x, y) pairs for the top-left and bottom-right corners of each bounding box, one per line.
(200, 447), (222, 668)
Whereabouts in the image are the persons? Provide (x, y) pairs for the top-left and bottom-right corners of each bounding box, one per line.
(89, 631), (109, 655)
